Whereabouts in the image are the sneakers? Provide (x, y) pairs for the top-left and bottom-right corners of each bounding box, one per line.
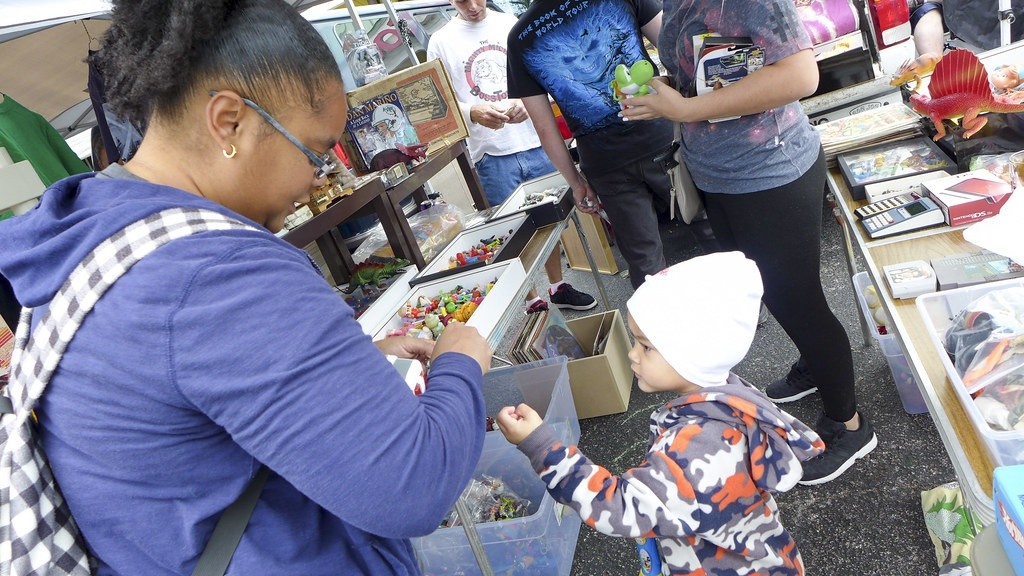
(527, 299), (549, 313)
(796, 411), (880, 480)
(765, 361), (818, 403)
(548, 282), (597, 310)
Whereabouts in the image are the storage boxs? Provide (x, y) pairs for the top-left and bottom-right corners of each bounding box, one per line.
(338, 57), (470, 171)
(920, 168), (1013, 227)
(916, 276), (1024, 576)
(837, 136), (958, 201)
(851, 272), (929, 415)
(332, 164), (634, 576)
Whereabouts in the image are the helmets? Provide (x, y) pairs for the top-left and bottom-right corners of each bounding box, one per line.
(372, 103), (403, 131)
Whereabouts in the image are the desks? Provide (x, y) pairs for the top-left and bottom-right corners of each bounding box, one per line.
(824, 168), (999, 527)
(278, 140), (490, 285)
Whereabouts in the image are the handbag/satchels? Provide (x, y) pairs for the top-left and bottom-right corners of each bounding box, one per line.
(666, 144), (700, 225)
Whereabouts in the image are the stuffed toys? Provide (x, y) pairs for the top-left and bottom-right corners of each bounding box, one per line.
(611, 61), (658, 110)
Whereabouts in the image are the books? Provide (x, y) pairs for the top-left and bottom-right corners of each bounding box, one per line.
(810, 99), (925, 169)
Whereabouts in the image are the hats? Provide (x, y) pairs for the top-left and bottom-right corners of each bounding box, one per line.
(626, 251), (765, 385)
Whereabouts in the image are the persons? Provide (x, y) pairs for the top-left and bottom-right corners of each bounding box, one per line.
(504, 1), (769, 327)
(619, 0), (878, 485)
(357, 127), (366, 141)
(496, 250), (826, 576)
(907, 0), (943, 64)
(371, 103), (404, 140)
(0, 0), (494, 576)
(425, 0), (598, 316)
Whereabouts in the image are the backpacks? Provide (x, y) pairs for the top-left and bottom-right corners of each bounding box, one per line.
(0, 206), (277, 576)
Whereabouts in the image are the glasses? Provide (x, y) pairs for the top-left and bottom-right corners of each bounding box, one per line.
(208, 90), (337, 178)
